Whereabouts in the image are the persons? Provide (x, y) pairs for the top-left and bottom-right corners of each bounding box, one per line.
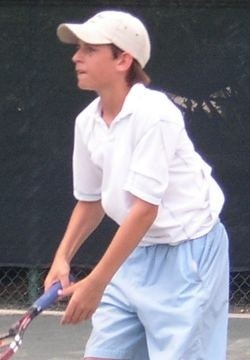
(43, 9), (230, 360)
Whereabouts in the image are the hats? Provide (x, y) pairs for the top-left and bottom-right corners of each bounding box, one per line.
(56, 10), (151, 69)
